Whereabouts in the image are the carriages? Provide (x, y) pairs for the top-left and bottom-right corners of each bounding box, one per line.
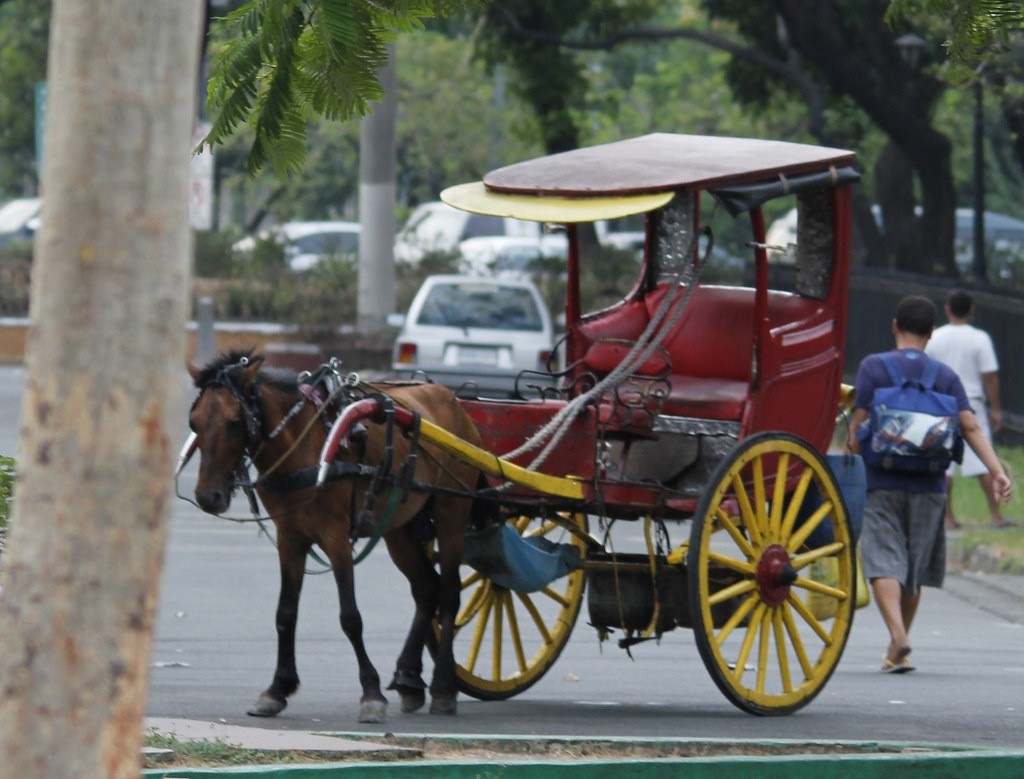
(188, 132), (863, 725)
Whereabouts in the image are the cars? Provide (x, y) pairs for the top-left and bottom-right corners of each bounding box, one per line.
(387, 275), (566, 399)
(764, 205), (1024, 272)
(395, 201), (747, 274)
(232, 221), (359, 272)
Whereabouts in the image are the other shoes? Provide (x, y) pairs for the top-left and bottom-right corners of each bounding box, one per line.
(985, 520), (1017, 529)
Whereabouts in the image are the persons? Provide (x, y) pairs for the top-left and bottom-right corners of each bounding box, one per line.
(846, 288), (1013, 672)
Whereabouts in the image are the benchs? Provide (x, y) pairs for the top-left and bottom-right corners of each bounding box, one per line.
(577, 277), (803, 420)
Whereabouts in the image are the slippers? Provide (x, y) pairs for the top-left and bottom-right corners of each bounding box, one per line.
(878, 652), (915, 674)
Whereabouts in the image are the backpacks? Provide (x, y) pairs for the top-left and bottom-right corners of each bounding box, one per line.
(860, 352), (958, 476)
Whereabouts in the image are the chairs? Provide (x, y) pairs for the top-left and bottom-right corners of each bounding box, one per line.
(511, 336), (671, 439)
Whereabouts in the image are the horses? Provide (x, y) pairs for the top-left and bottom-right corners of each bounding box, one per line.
(186, 346), (503, 724)
